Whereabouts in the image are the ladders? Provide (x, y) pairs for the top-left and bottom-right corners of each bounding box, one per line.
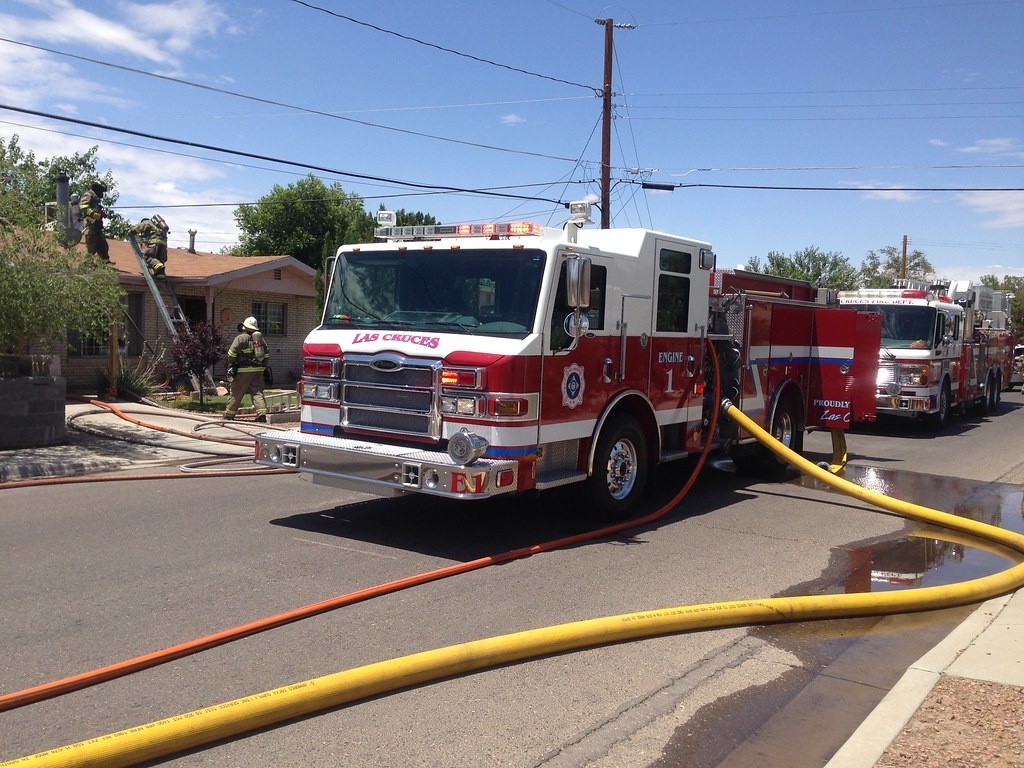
(128, 233), (219, 396)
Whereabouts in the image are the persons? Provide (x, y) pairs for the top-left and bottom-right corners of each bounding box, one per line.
(128, 217), (173, 276)
(223, 316), (269, 421)
(79, 181), (109, 264)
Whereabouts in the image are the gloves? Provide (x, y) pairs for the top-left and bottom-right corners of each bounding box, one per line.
(263, 369), (270, 378)
(227, 367), (237, 378)
(92, 213), (101, 220)
(124, 233), (131, 240)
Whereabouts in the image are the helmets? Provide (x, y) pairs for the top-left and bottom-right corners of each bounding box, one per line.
(141, 217), (149, 222)
(242, 316), (259, 330)
(91, 180), (107, 198)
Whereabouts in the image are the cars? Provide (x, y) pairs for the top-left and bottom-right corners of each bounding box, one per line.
(1009, 343), (1024, 389)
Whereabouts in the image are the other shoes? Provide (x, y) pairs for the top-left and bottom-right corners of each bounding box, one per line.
(153, 262), (165, 275)
(254, 415), (267, 422)
(223, 413), (235, 420)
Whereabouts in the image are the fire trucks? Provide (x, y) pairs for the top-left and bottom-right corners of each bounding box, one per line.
(871, 536), (968, 588)
(250, 201), (884, 520)
(832, 274), (1018, 427)
